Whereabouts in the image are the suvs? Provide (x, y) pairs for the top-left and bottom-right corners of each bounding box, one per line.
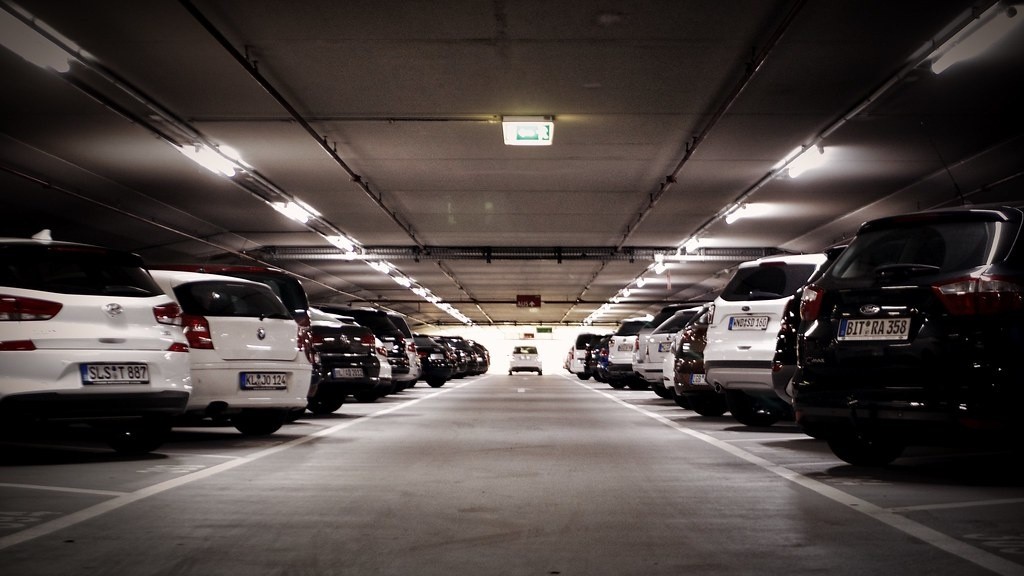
(570, 317), (653, 389)
(773, 205), (1023, 476)
(0, 229), (193, 448)
(139, 266), (319, 442)
(312, 303), (421, 416)
(417, 335), (488, 387)
(634, 255), (827, 421)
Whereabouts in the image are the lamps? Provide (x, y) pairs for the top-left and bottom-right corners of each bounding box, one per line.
(1, 0), (474, 341)
(584, 0), (1024, 326)
(502, 116), (555, 145)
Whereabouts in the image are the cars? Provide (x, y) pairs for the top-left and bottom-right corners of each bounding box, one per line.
(509, 345), (545, 375)
